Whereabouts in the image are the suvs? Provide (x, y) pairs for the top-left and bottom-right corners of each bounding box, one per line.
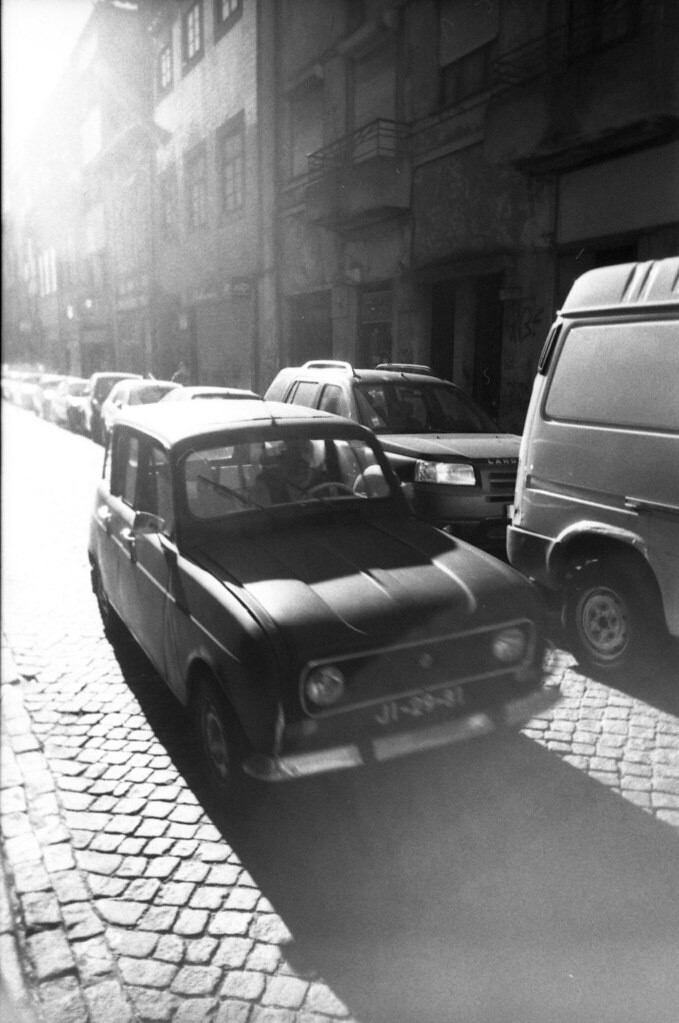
(266, 357), (524, 544)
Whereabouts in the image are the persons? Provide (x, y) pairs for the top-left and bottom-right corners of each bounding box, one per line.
(274, 438), (338, 505)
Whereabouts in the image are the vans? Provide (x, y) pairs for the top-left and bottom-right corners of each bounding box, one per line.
(501, 260), (679, 677)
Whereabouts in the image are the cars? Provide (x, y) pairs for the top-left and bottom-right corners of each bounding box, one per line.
(84, 397), (566, 804)
(2, 364), (274, 484)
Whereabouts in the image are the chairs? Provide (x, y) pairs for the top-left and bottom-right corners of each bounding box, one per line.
(157, 457), (230, 527)
(386, 400), (423, 434)
